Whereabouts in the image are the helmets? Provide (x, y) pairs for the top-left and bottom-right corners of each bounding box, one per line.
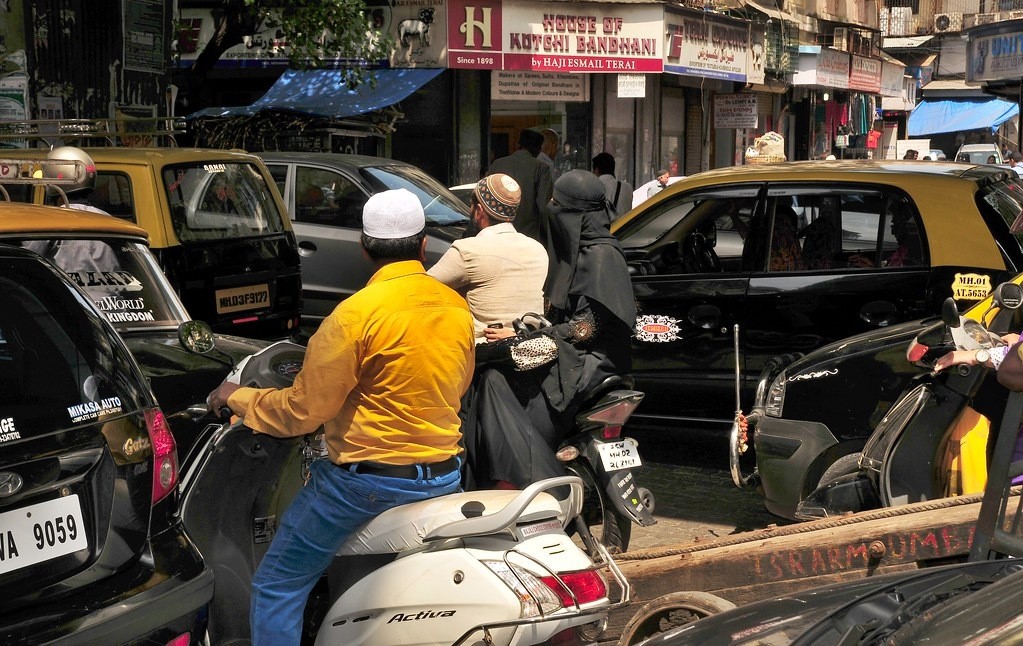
(37, 146), (98, 197)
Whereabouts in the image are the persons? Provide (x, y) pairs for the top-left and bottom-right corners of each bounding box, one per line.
(486, 129), (550, 240)
(934, 329), (1023, 489)
(903, 150), (918, 160)
(987, 155), (996, 164)
(1001, 143), (1012, 160)
(207, 188), (474, 646)
(538, 128), (560, 180)
(464, 169), (635, 490)
(21, 146), (123, 299)
(592, 152), (633, 223)
(427, 172), (549, 345)
(849, 202), (919, 268)
(923, 156), (931, 160)
(1009, 151), (1023, 180)
(647, 170), (669, 199)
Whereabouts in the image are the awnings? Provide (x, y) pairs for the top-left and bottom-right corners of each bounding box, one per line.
(181, 65), (447, 118)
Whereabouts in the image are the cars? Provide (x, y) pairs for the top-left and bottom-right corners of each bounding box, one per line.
(1, 145), (1023, 646)
(0, 244), (217, 646)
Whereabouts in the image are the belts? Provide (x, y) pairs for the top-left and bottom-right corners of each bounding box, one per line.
(337, 456), (459, 481)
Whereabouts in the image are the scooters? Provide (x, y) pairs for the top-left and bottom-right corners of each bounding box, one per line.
(165, 318), (632, 646)
(549, 369), (659, 559)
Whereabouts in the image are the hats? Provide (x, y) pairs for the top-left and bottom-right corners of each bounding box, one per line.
(552, 169), (607, 212)
(654, 170), (668, 179)
(519, 128), (545, 148)
(82, 374), (103, 403)
(476, 173), (522, 221)
(362, 187), (425, 240)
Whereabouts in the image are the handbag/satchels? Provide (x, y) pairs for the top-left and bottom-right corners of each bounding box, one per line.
(474, 312), (559, 373)
(939, 405), (991, 497)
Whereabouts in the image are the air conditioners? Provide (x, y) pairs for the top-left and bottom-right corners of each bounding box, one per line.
(834, 27), (861, 53)
(933, 11), (963, 33)
(858, 37), (871, 58)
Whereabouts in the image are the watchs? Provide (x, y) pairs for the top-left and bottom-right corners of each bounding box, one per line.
(976, 350), (989, 368)
